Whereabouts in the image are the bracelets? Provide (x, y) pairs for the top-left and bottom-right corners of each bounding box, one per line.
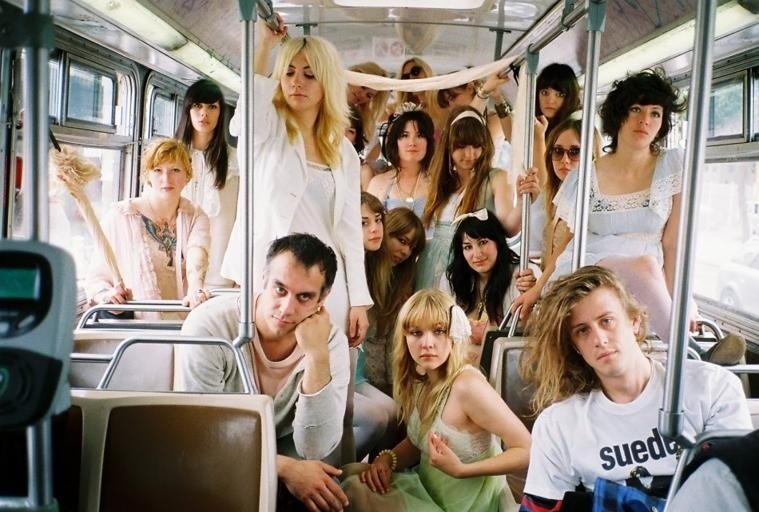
(375, 449), (399, 474)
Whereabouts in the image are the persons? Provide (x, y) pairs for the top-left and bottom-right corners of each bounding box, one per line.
(170, 80), (241, 290)
(86, 137), (214, 322)
(669, 431), (758, 511)
(220, 12), (374, 465)
(333, 287), (532, 511)
(339, 57), (601, 457)
(509, 70), (748, 365)
(517, 264), (756, 511)
(178, 232), (351, 509)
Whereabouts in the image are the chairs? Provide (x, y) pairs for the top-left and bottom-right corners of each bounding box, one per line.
(68, 328), (173, 390)
(51, 389), (277, 511)
(489, 335), (750, 453)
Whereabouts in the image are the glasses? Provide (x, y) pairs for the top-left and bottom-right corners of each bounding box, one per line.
(549, 146), (580, 161)
(401, 66), (421, 79)
